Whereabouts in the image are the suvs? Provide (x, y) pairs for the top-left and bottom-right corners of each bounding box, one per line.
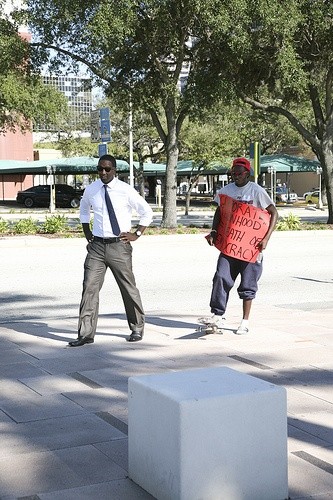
(303, 188), (320, 199)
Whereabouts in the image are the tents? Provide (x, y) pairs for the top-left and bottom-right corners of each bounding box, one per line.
(0, 154), (324, 214)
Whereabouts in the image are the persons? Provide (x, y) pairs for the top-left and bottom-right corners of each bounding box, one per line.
(197, 158), (278, 334)
(69, 154), (154, 346)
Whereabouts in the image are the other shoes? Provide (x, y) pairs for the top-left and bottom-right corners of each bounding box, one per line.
(236, 325), (249, 334)
(200, 316), (225, 329)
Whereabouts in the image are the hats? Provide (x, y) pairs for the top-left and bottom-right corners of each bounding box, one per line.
(232, 158), (250, 171)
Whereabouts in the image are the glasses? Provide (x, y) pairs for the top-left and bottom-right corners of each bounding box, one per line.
(231, 170), (247, 177)
(97, 166), (114, 172)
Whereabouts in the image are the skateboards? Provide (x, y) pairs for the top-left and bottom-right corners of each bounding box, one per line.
(196, 317), (226, 334)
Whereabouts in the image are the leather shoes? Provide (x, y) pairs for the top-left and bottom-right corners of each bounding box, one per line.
(69, 336), (94, 346)
(128, 331), (143, 341)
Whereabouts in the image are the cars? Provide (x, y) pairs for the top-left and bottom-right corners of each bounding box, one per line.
(16, 183), (81, 208)
(267, 186), (297, 204)
(305, 191), (320, 204)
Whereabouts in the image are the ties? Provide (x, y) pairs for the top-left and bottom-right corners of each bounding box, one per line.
(103, 185), (120, 236)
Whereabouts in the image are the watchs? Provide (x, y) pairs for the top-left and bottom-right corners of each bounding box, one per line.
(135, 230), (141, 237)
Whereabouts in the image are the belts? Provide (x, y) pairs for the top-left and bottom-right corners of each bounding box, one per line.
(94, 236), (119, 244)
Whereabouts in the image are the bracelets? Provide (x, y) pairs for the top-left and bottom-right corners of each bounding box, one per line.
(211, 230), (217, 233)
